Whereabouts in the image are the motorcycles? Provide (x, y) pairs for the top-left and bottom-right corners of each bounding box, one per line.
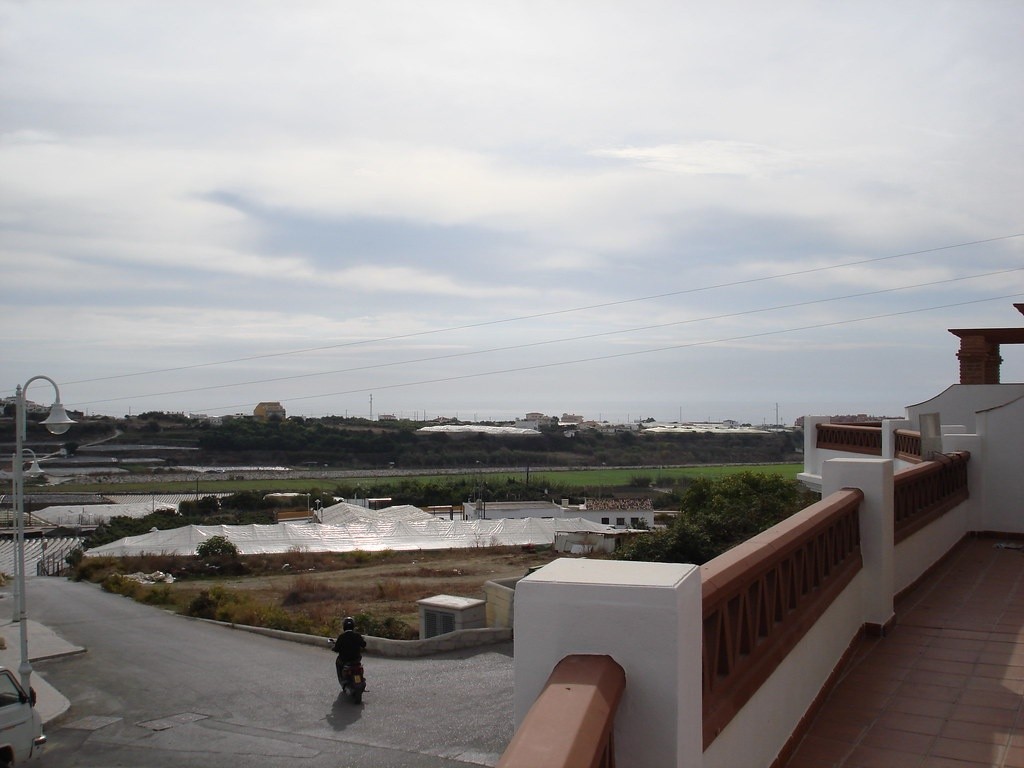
(327, 635), (371, 705)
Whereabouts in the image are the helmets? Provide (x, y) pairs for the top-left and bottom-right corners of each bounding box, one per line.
(343, 617), (355, 631)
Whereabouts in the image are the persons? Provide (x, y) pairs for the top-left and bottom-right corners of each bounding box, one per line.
(334, 616), (366, 681)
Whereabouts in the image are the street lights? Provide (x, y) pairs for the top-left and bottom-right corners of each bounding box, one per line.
(195, 470), (205, 503)
(9, 446), (45, 623)
(314, 498), (321, 523)
(11, 375), (81, 701)
(320, 463), (329, 498)
(306, 492), (311, 524)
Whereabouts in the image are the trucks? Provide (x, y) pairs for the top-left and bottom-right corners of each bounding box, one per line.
(0, 665), (47, 768)
